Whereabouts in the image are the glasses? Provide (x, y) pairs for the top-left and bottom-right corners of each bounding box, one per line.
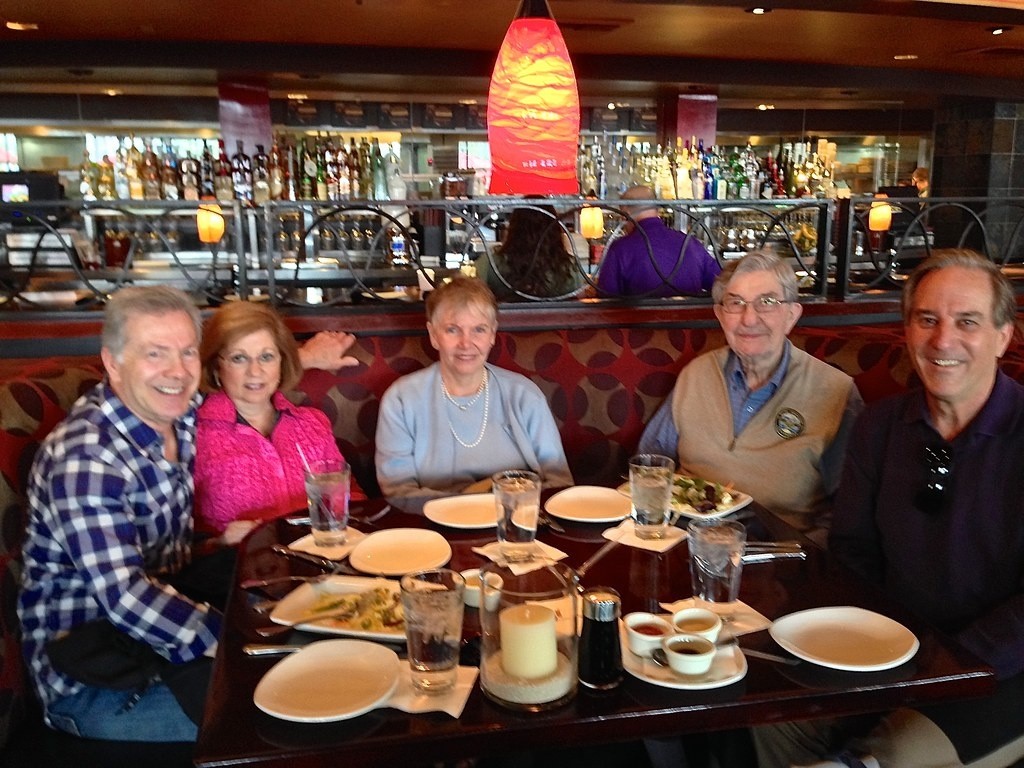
(215, 352), (282, 367)
(719, 293), (792, 313)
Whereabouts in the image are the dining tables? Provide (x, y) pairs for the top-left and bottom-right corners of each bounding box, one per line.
(191, 476), (997, 768)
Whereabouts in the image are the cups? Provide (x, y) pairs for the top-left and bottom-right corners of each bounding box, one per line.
(303, 457), (352, 547)
(477, 555), (582, 712)
(398, 569), (465, 698)
(628, 453), (675, 541)
(492, 469), (542, 544)
(686, 516), (748, 623)
(416, 268), (435, 291)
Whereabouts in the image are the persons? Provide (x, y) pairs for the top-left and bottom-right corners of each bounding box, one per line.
(596, 186), (722, 298)
(750, 246), (1024, 768)
(193, 300), (368, 557)
(636, 250), (863, 549)
(376, 274), (576, 517)
(17, 285), (359, 741)
(477, 195), (582, 303)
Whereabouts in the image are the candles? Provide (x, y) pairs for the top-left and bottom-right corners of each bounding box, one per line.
(498, 604), (558, 679)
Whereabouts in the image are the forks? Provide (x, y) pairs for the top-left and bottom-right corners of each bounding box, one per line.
(286, 505), (392, 524)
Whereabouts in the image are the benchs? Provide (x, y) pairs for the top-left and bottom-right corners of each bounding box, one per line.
(0, 319), (1024, 768)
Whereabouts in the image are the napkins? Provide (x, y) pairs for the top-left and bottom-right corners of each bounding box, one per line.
(657, 594), (774, 643)
(287, 524), (370, 561)
(602, 515), (692, 554)
(376, 659), (479, 717)
(470, 538), (570, 575)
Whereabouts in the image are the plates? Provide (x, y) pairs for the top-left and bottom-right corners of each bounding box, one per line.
(618, 473), (753, 522)
(510, 502), (539, 532)
(767, 606), (921, 672)
(544, 485), (633, 522)
(349, 526), (453, 576)
(423, 492), (506, 529)
(252, 638), (401, 724)
(269, 573), (449, 644)
(614, 615), (749, 690)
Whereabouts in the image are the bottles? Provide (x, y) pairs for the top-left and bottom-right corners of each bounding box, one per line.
(575, 587), (625, 690)
(78, 131), (848, 267)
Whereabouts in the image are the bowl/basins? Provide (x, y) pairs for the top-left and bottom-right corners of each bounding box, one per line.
(622, 612), (673, 657)
(673, 607), (723, 643)
(455, 569), (504, 608)
(661, 634), (717, 675)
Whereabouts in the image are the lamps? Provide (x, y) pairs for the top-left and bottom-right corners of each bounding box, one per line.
(485, 0), (581, 195)
(869, 192), (893, 232)
(580, 190), (604, 240)
(197, 188), (225, 243)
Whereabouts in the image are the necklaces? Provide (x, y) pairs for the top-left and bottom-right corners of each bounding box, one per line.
(441, 367), (489, 448)
